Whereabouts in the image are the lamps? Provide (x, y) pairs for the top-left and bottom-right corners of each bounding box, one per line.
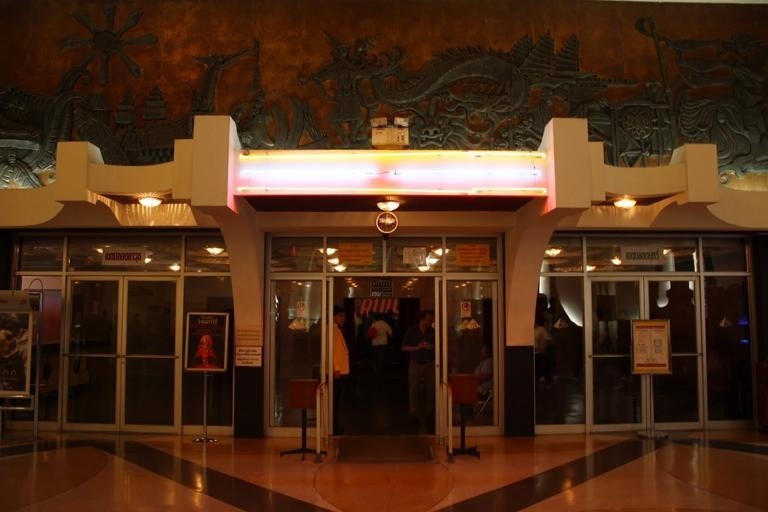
(138, 197), (163, 208)
(613, 198), (637, 210)
(416, 246), (451, 273)
(376, 201), (401, 212)
(204, 246), (225, 255)
(318, 247), (348, 274)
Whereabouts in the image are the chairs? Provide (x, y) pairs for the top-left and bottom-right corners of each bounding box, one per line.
(448, 372), (483, 458)
(280, 378), (328, 457)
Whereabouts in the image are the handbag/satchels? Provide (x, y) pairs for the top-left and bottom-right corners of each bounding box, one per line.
(366, 326), (378, 339)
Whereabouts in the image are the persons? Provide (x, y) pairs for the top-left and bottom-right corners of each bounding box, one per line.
(533, 318), (550, 384)
(470, 344), (493, 401)
(327, 306), (435, 436)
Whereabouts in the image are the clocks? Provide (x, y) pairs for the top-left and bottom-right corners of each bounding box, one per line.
(376, 212), (399, 235)
